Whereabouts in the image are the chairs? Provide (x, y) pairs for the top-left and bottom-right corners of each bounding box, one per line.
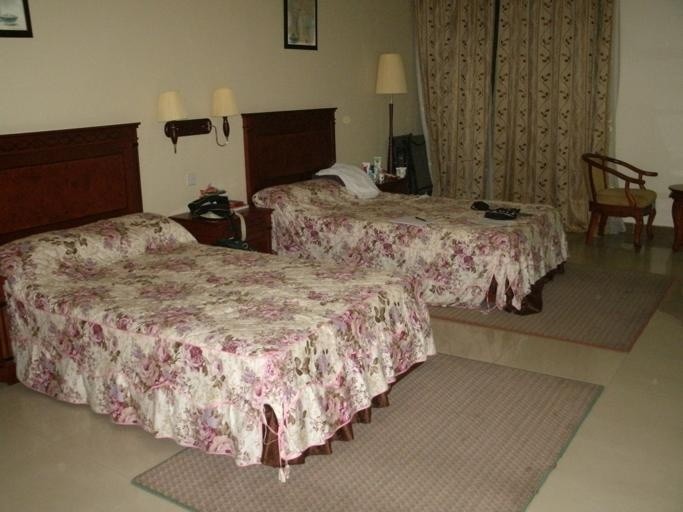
(578, 148), (659, 253)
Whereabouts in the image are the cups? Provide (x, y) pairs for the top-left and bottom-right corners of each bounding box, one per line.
(396, 167), (407, 179)
(362, 157), (384, 184)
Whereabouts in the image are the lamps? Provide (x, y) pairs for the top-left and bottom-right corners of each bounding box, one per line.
(373, 50), (409, 173)
(154, 88), (240, 155)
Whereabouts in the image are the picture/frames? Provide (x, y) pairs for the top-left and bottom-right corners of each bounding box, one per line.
(0, 1), (35, 40)
(280, 0), (320, 51)
